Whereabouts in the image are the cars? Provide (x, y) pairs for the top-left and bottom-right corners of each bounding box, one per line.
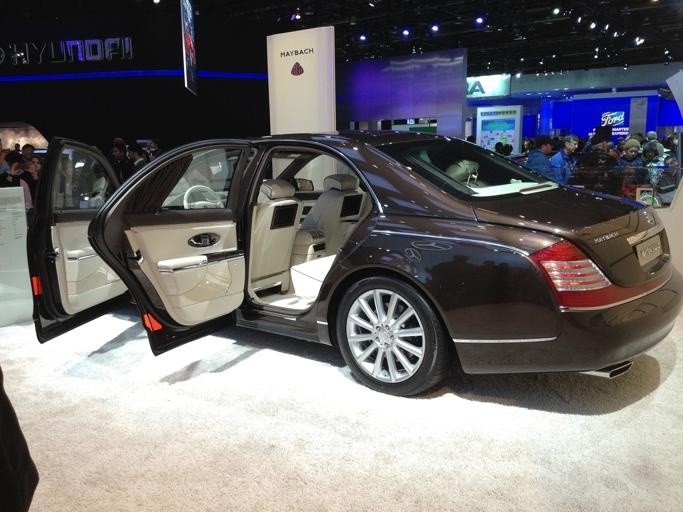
(126, 139), (159, 159)
(25, 125), (682, 394)
(502, 147), (650, 195)
(10, 143), (80, 181)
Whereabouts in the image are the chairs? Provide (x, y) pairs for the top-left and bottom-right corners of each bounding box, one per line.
(300, 172), (367, 256)
(249, 178), (303, 292)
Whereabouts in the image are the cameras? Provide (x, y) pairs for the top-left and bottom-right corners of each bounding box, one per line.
(18, 164), (29, 169)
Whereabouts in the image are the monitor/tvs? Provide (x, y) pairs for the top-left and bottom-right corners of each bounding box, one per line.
(340, 194), (363, 217)
(270, 204), (299, 229)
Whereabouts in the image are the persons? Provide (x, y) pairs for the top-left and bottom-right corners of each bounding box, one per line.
(1, 137), (164, 211)
(467, 126), (683, 197)
